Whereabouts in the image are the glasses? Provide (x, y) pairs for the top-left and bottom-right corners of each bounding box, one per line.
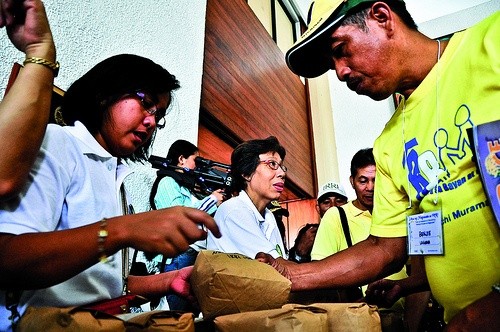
(258, 158), (287, 172)
(136, 93), (166, 127)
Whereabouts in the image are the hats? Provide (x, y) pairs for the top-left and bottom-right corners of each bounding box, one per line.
(317, 182), (348, 201)
(286, 0), (405, 78)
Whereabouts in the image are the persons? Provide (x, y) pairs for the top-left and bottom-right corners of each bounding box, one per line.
(255, 0), (500, 332)
(206, 135), (288, 260)
(311, 147), (444, 332)
(0, 53), (222, 332)
(289, 181), (348, 264)
(154, 139), (227, 311)
(0, 1), (60, 203)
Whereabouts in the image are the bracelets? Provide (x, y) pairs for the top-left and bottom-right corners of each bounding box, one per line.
(22, 56), (60, 77)
(294, 251), (308, 262)
(98, 216), (109, 264)
